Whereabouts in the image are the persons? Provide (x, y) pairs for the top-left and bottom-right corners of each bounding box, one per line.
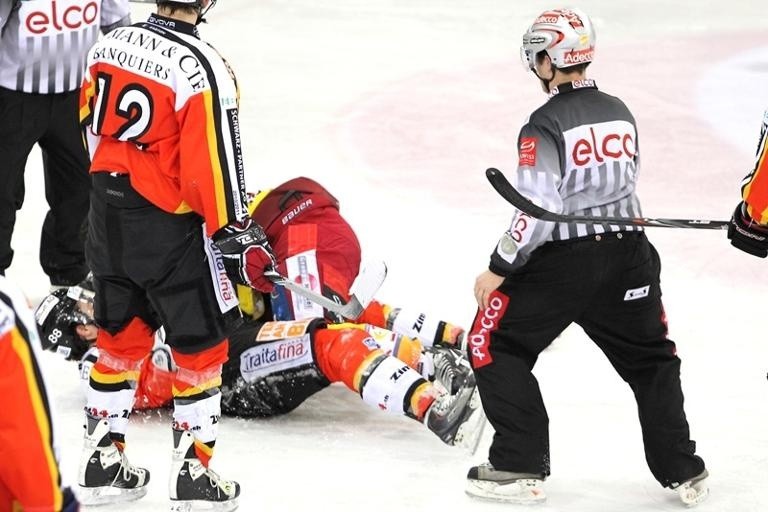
(78, 0), (277, 502)
(35, 285), (477, 447)
(466, 7), (708, 492)
(0, 0), (131, 290)
(0, 289), (81, 512)
(236, 177), (466, 349)
(728, 108), (768, 258)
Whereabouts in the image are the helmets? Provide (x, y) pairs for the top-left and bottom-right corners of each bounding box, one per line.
(518, 7), (597, 72)
(34, 285), (96, 360)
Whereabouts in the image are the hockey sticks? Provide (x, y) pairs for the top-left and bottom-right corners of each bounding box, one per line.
(486, 167), (731, 230)
(263, 261), (387, 320)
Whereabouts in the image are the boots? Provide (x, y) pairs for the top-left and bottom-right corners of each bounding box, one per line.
(77, 413), (150, 492)
(404, 330), (477, 446)
(170, 423), (240, 502)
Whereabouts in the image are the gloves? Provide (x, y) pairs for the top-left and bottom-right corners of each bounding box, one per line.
(728, 201), (768, 258)
(210, 217), (278, 294)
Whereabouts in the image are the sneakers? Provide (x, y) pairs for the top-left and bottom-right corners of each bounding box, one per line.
(669, 454), (709, 488)
(468, 461), (545, 483)
